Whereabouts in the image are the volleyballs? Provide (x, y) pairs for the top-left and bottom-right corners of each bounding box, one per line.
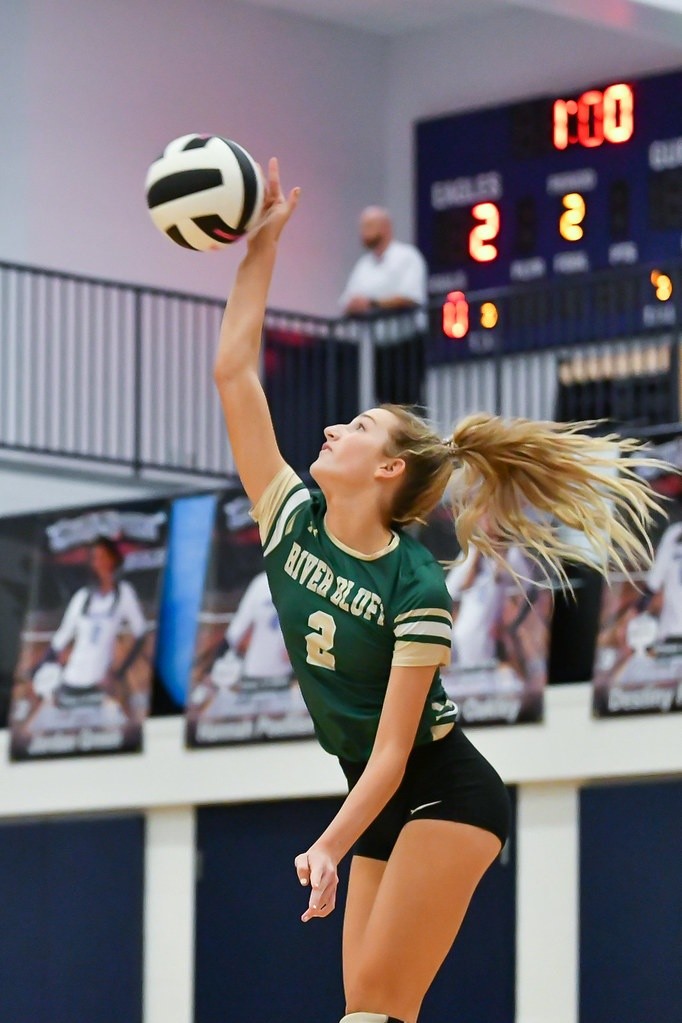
(144, 133), (264, 252)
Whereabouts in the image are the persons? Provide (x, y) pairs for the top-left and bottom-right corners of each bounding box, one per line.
(439, 513), (533, 722)
(191, 572), (295, 742)
(12, 539), (147, 755)
(212, 158), (682, 1023)
(336, 205), (430, 420)
(605, 522), (682, 712)
